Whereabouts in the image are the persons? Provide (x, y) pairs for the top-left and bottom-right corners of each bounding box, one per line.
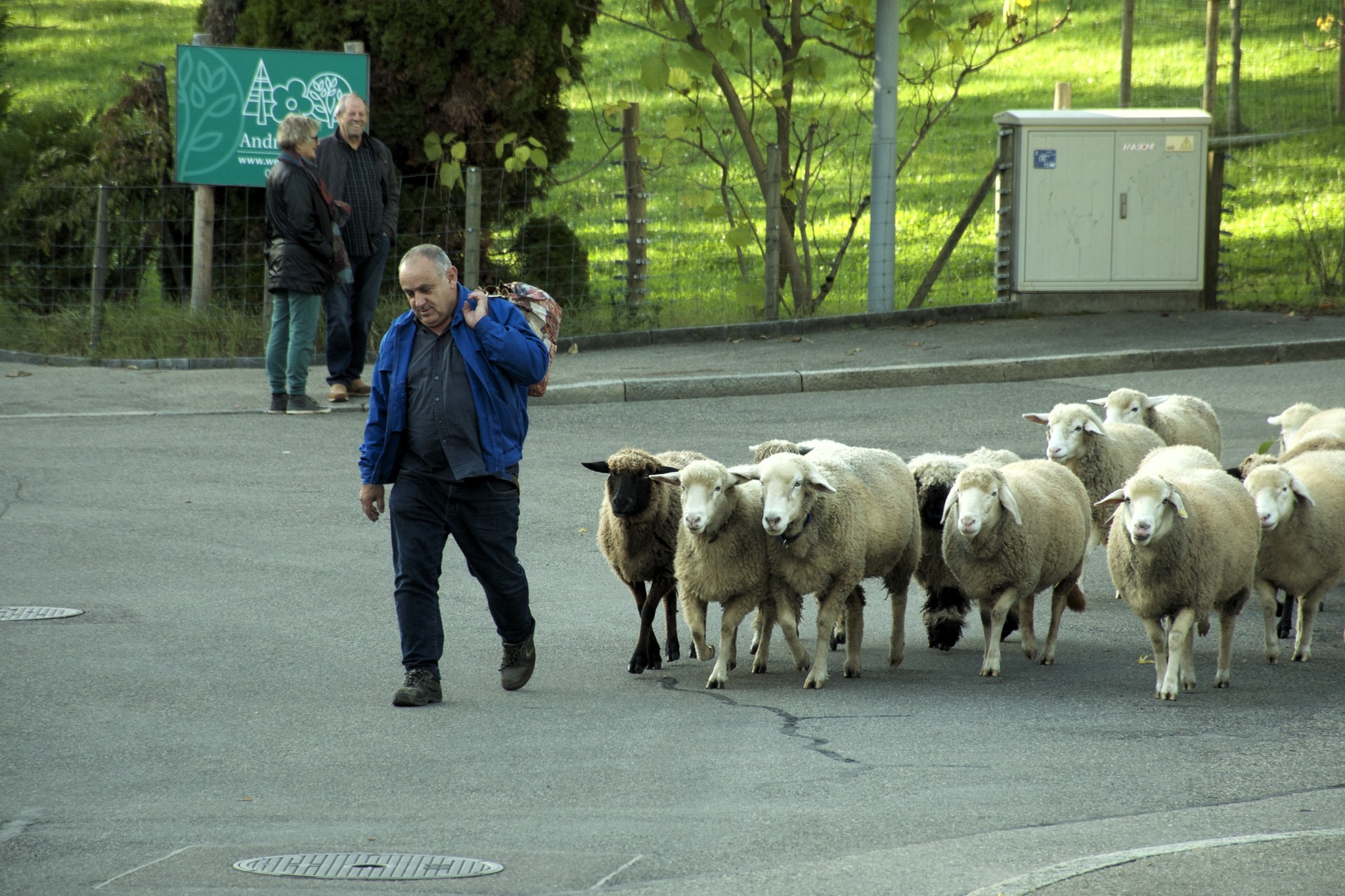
(359, 243), (550, 707)
(263, 113), (354, 416)
(308, 94), (400, 404)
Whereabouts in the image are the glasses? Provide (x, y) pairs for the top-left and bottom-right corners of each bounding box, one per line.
(310, 136), (318, 143)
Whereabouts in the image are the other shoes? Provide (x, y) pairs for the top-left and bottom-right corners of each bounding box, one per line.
(349, 378), (371, 396)
(329, 382), (349, 402)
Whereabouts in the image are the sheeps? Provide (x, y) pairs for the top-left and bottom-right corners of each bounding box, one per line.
(582, 385), (1344, 699)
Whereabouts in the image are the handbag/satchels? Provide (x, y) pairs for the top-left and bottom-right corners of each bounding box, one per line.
(478, 280), (563, 397)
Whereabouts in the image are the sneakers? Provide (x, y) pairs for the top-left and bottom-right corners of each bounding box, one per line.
(286, 393), (332, 415)
(268, 392), (289, 414)
(498, 633), (537, 691)
(393, 668), (442, 706)
